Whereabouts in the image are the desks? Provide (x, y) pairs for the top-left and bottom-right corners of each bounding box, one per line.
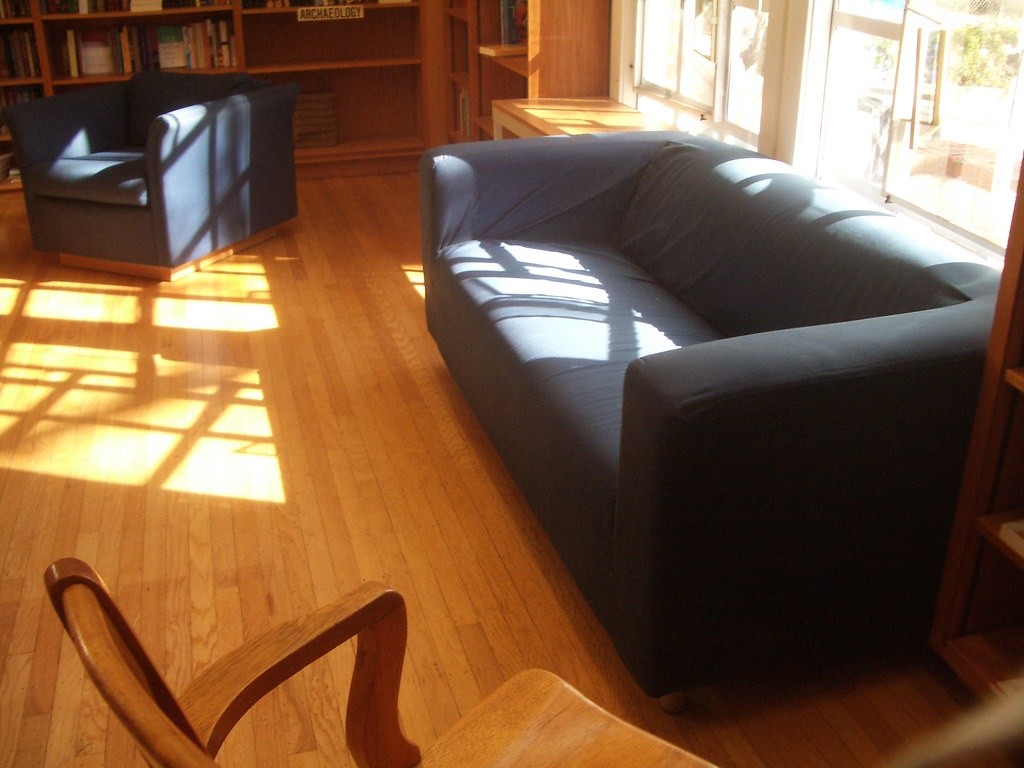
(491, 96), (679, 144)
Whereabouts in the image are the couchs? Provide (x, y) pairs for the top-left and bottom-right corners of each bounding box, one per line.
(419, 131), (1010, 717)
(0, 71), (300, 283)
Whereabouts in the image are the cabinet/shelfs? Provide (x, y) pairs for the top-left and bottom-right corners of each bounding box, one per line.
(0, 0), (612, 194)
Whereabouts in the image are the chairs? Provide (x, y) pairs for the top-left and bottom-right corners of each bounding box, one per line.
(43, 556), (721, 768)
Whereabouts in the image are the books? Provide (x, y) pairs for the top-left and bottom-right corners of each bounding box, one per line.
(478, 0), (528, 57)
(50, 18), (234, 78)
(240, 0), (412, 10)
(0, 0), (233, 19)
(0, 26), (40, 80)
(0, 84), (43, 184)
(459, 90), (470, 135)
